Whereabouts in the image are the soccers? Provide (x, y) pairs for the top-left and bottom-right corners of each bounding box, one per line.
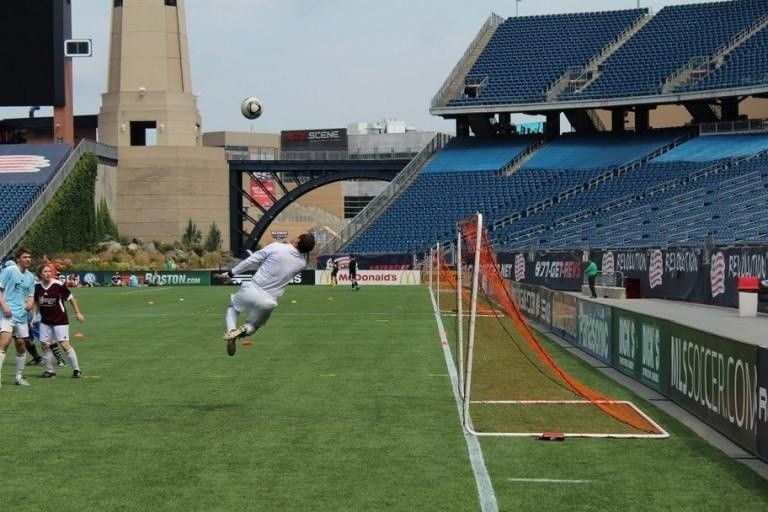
(240, 96), (264, 120)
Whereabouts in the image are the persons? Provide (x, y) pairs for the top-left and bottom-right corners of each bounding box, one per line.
(0, 247), (35, 386)
(111, 272), (122, 286)
(128, 272), (139, 286)
(4, 260), (66, 367)
(585, 257), (598, 298)
(57, 272), (79, 287)
(348, 254), (360, 291)
(211, 233), (314, 356)
(329, 261), (338, 286)
(43, 255), (57, 278)
(28, 264), (85, 379)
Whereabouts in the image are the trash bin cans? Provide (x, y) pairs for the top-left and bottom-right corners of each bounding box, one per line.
(737, 276), (760, 317)
(626, 278), (640, 298)
(129, 275), (139, 287)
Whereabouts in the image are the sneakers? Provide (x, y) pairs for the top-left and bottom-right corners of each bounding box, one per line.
(329, 281), (361, 292)
(23, 356), (43, 367)
(14, 377), (30, 387)
(221, 324), (248, 342)
(70, 369), (82, 379)
(40, 371), (57, 379)
(225, 329), (238, 356)
(56, 359), (66, 368)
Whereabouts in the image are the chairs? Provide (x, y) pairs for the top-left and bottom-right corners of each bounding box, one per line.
(0, 143), (70, 242)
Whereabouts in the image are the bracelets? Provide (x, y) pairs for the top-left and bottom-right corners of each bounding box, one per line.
(76, 311), (80, 314)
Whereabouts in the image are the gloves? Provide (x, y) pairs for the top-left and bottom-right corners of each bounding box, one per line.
(211, 271), (230, 281)
